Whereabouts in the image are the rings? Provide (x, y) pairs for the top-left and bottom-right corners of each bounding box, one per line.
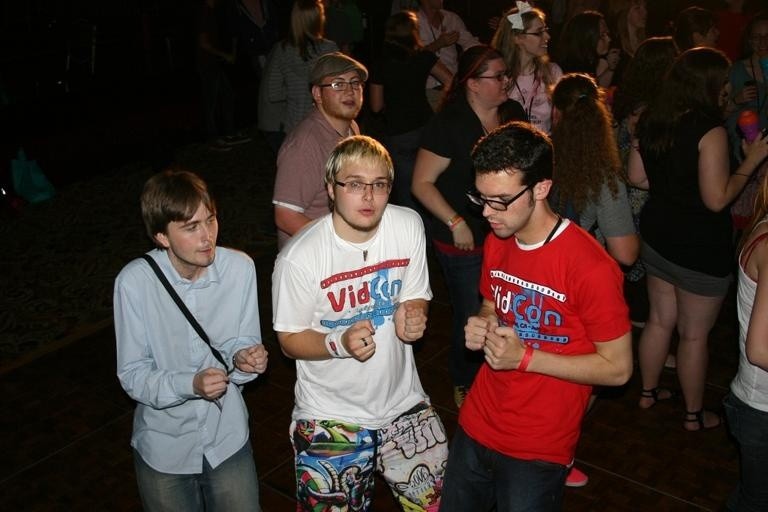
(361, 337), (368, 346)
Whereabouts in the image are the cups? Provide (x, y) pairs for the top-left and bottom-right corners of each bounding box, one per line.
(737, 110), (759, 144)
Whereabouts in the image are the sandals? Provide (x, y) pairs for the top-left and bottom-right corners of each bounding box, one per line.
(680, 407), (727, 433)
(638, 386), (679, 408)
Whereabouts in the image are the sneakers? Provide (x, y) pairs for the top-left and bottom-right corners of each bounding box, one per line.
(453, 384), (469, 407)
(567, 466), (587, 486)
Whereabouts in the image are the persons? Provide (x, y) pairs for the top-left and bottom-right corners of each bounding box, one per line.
(410, 45), (531, 370)
(492, 1), (564, 137)
(271, 135), (449, 512)
(442, 121), (634, 511)
(611, 0), (648, 55)
(265, 0), (338, 126)
(625, 36), (678, 282)
(672, 6), (723, 52)
(273, 51), (368, 249)
(629, 46), (768, 434)
(371, 9), (452, 150)
(112, 170), (269, 512)
(547, 74), (639, 488)
(723, 167), (768, 512)
(562, 10), (619, 88)
(411, 0), (477, 113)
(727, 21), (768, 170)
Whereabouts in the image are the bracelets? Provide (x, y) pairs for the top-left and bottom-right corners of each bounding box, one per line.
(325, 334), (351, 358)
(447, 215), (466, 231)
(517, 346), (534, 370)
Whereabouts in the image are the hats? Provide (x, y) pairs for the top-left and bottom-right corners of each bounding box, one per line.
(308, 51), (369, 85)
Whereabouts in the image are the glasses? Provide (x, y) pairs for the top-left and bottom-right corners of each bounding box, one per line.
(335, 179), (393, 196)
(318, 81), (365, 90)
(465, 183), (534, 211)
(523, 28), (550, 36)
(478, 69), (513, 82)
